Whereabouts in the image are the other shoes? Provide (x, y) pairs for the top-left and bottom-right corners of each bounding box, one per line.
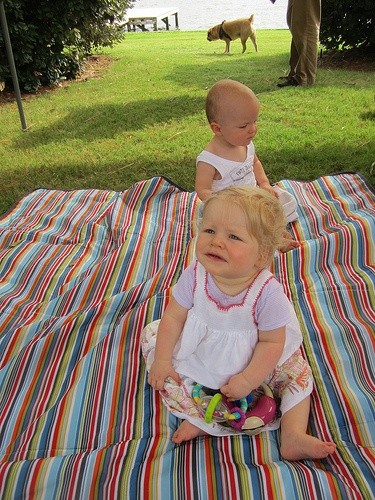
(278, 76), (293, 80)
(277, 79), (299, 87)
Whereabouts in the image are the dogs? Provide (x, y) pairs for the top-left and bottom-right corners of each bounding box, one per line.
(207, 14), (258, 54)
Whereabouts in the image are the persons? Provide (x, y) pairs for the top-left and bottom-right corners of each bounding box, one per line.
(139, 186), (337, 460)
(277, 0), (321, 87)
(196, 79), (300, 253)
